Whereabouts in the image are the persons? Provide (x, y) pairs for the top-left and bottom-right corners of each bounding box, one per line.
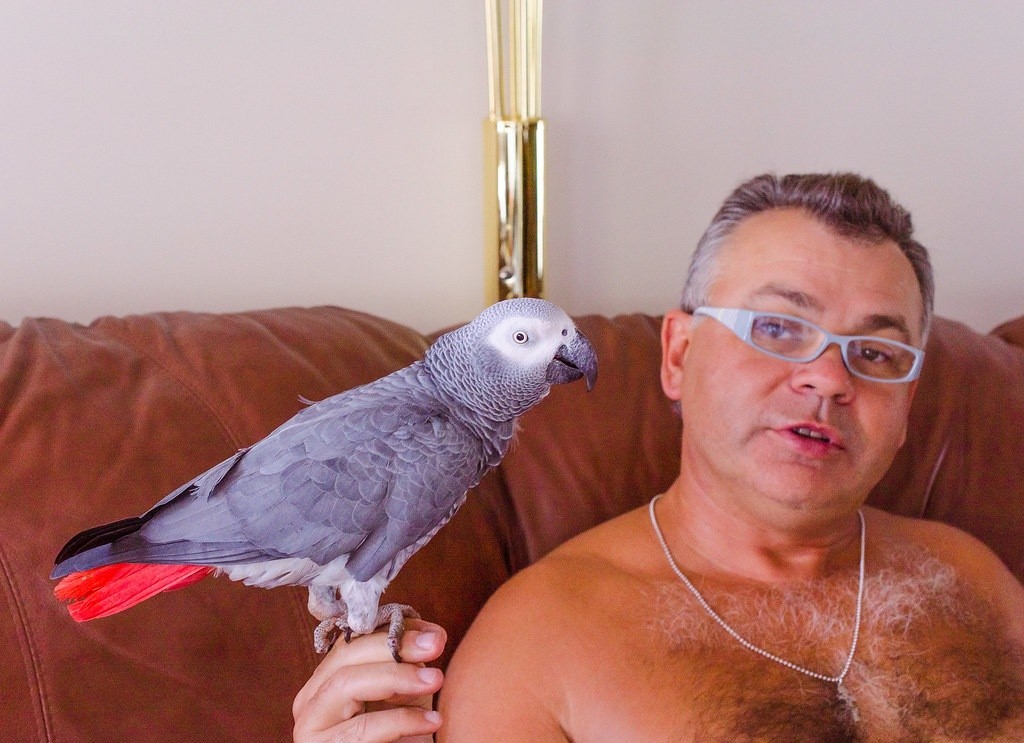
(290, 172), (1024, 743)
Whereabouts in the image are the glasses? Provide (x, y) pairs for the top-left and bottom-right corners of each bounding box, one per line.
(693, 306), (925, 383)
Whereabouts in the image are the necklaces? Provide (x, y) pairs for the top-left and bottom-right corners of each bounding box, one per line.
(649, 493), (866, 719)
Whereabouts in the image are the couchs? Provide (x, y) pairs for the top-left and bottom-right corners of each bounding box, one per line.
(0, 305), (1024, 743)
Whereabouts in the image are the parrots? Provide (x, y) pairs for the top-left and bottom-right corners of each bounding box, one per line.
(51, 297), (599, 664)
